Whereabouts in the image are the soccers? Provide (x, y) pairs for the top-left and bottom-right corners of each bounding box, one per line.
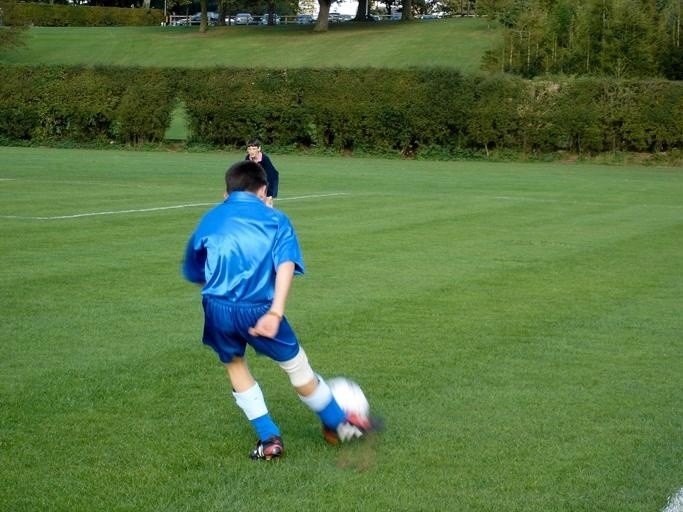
(320, 375), (370, 417)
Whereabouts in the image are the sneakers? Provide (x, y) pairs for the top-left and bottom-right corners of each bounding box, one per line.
(249, 436), (284, 462)
(324, 412), (372, 446)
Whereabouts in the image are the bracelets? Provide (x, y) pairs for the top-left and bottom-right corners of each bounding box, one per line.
(266, 311), (282, 321)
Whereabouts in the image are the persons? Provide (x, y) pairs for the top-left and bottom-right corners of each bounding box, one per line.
(245, 139), (279, 208)
(181, 161), (368, 461)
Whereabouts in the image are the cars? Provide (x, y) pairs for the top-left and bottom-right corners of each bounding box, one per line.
(328, 13), (339, 22)
(260, 14), (280, 25)
(234, 14), (253, 24)
(366, 14), (379, 21)
(392, 12), (401, 20)
(421, 14), (436, 20)
(190, 11), (218, 25)
(336, 16), (351, 22)
